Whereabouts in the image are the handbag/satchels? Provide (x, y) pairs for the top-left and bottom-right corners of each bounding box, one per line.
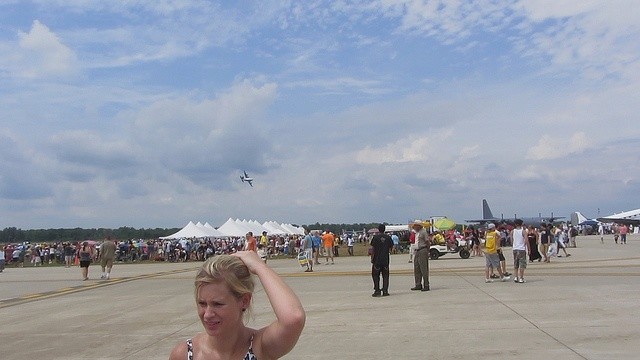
(312, 245), (316, 253)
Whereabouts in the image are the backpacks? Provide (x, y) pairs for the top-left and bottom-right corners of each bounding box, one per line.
(571, 227), (578, 236)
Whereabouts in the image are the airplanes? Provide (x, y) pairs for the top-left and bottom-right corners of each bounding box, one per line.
(575, 209), (640, 226)
(240, 170), (254, 187)
(464, 198), (566, 227)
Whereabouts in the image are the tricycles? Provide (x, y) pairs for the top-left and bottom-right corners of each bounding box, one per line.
(430, 238), (470, 259)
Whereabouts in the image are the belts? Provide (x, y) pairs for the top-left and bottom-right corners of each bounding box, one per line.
(415, 246), (427, 251)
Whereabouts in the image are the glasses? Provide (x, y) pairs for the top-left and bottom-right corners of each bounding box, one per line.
(528, 227), (531, 228)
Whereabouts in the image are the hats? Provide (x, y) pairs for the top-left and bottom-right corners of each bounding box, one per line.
(487, 223), (495, 230)
(348, 234), (352, 236)
(409, 220), (432, 227)
(305, 226), (310, 232)
(262, 231), (267, 234)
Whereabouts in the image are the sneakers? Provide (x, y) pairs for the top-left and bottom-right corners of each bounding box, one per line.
(305, 269), (310, 271)
(82, 277), (86, 281)
(421, 287), (430, 291)
(501, 276), (510, 282)
(513, 277), (518, 282)
(85, 277), (89, 279)
(101, 275), (106, 278)
(556, 254), (561, 257)
(504, 272), (512, 276)
(485, 278), (495, 282)
(106, 276), (110, 279)
(490, 274), (500, 279)
(411, 285), (422, 290)
(566, 254), (570, 257)
(519, 278), (526, 283)
(308, 270), (313, 272)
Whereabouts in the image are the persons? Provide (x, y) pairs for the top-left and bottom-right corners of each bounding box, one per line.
(510, 219), (531, 282)
(256, 236), (261, 250)
(116, 236), (245, 263)
(260, 231), (267, 264)
(93, 241), (102, 261)
(527, 224), (542, 262)
(619, 223), (627, 244)
(99, 236), (116, 280)
(312, 231), (321, 264)
(547, 224), (556, 258)
(390, 234), (399, 254)
(628, 224), (633, 235)
(79, 242), (92, 280)
(490, 223), (512, 279)
(397, 230), (410, 253)
(267, 235), (304, 257)
(410, 220), (432, 291)
(247, 232), (256, 251)
(598, 223), (604, 243)
(484, 222), (512, 282)
(355, 232), (373, 243)
(347, 234), (355, 255)
(577, 224), (598, 234)
(0, 240), (79, 271)
(538, 223), (550, 263)
(604, 223), (612, 234)
(334, 235), (339, 255)
(432, 227), (482, 255)
(303, 229), (314, 272)
(369, 225), (393, 297)
(340, 233), (347, 244)
(568, 224), (577, 248)
(496, 223), (513, 247)
(323, 229), (335, 264)
(612, 224), (619, 242)
(242, 233), (249, 250)
(167, 249), (306, 360)
(554, 223), (571, 257)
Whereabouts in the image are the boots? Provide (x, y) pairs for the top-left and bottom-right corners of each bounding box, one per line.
(383, 289), (389, 295)
(372, 290), (381, 297)
